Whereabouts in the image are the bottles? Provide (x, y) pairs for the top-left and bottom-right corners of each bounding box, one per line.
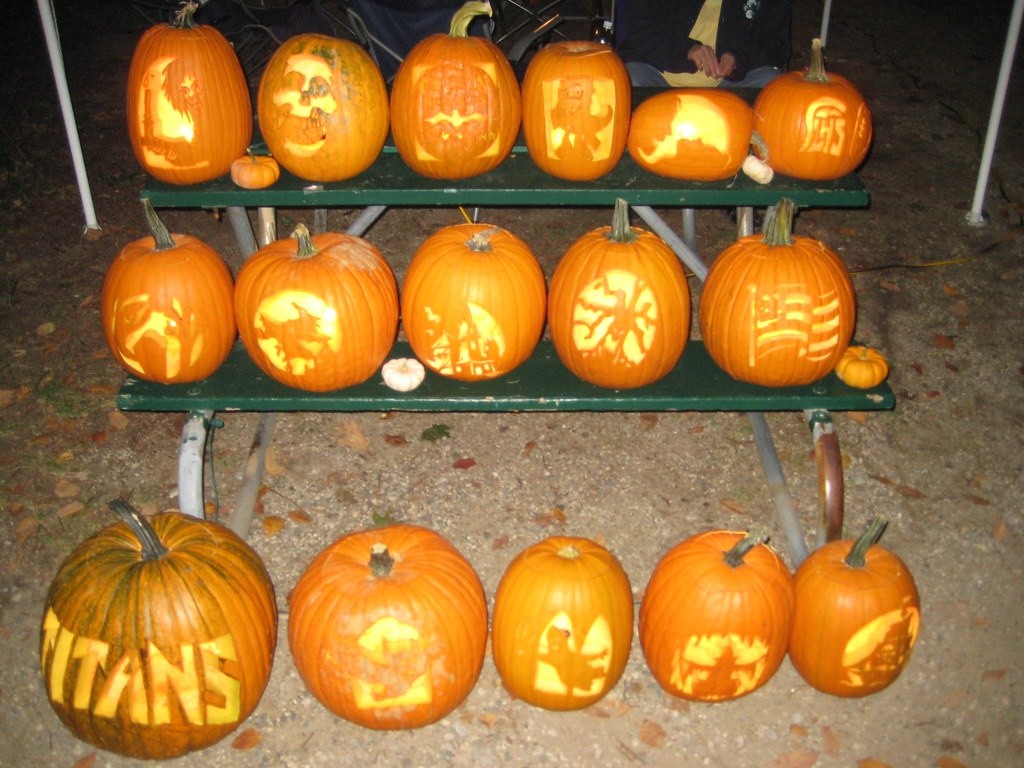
(593, 21), (615, 49)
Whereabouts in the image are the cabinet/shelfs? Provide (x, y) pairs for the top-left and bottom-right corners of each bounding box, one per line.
(117, 91), (897, 575)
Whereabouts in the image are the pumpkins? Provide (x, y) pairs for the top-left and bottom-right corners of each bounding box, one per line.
(231, 148), (279, 189)
(835, 345), (888, 388)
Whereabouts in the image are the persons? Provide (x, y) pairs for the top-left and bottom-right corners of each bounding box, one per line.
(612, 0), (791, 226)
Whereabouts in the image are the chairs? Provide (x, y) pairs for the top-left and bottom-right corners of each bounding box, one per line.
(115, 0), (576, 97)
